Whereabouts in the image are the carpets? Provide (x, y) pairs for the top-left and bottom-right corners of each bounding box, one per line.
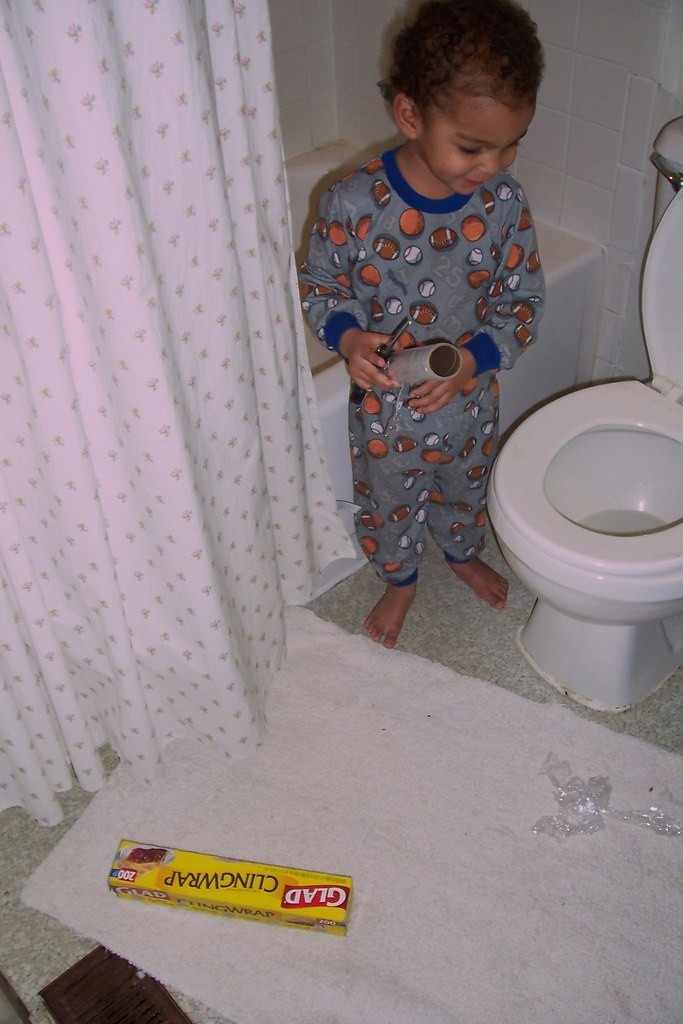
(18, 607), (683, 1024)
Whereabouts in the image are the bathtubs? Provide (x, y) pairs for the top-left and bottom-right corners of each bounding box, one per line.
(282, 134), (609, 614)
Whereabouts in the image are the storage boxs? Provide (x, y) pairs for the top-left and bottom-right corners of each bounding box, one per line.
(108, 839), (354, 937)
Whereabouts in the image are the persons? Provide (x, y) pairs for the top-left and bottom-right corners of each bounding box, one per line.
(299, 2), (545, 650)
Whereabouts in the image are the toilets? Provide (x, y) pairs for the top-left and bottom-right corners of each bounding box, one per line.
(486, 106), (682, 712)
